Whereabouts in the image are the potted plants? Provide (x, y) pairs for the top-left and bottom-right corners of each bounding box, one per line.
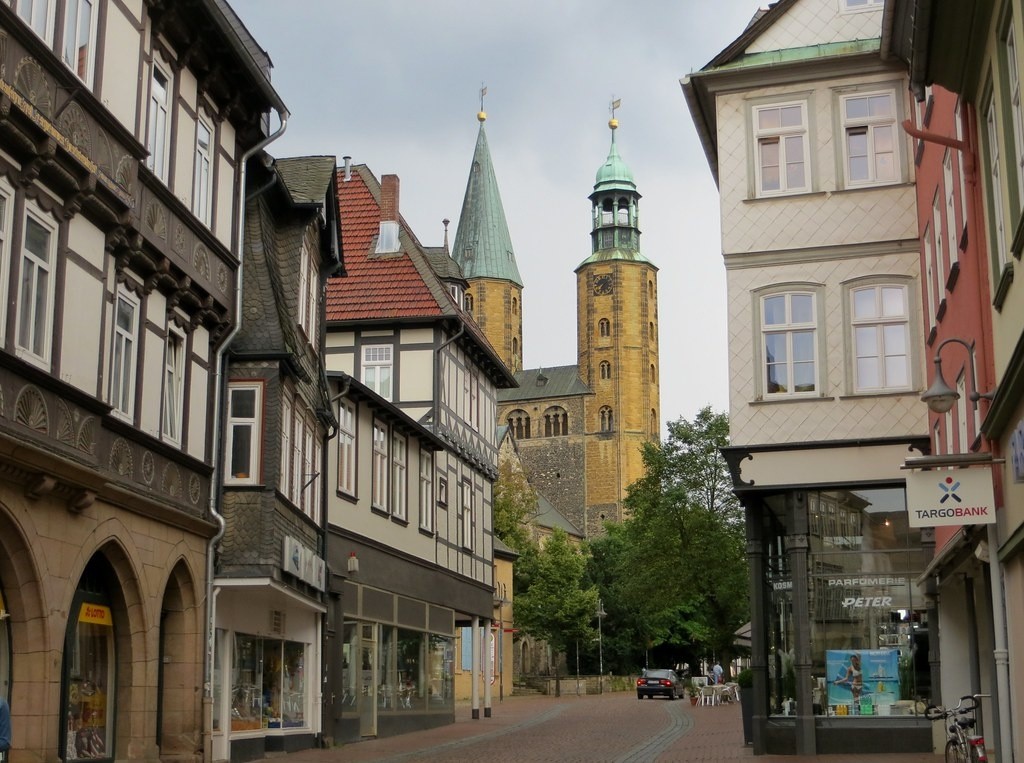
(689, 683), (698, 705)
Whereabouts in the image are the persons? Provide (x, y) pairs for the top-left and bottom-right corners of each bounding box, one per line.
(834, 654), (866, 703)
(712, 660), (725, 685)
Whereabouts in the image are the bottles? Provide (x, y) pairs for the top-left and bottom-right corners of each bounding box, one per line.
(878, 664), (886, 677)
(836, 692), (873, 715)
(878, 681), (886, 692)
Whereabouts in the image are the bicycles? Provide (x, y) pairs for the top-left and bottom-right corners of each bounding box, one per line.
(925, 694), (991, 763)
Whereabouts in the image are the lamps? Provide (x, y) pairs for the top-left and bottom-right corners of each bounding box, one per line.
(919, 335), (997, 413)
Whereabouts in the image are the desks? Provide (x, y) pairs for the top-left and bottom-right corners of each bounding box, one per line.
(704, 686), (727, 707)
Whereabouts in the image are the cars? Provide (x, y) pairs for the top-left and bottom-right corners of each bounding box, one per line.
(636, 668), (684, 700)
